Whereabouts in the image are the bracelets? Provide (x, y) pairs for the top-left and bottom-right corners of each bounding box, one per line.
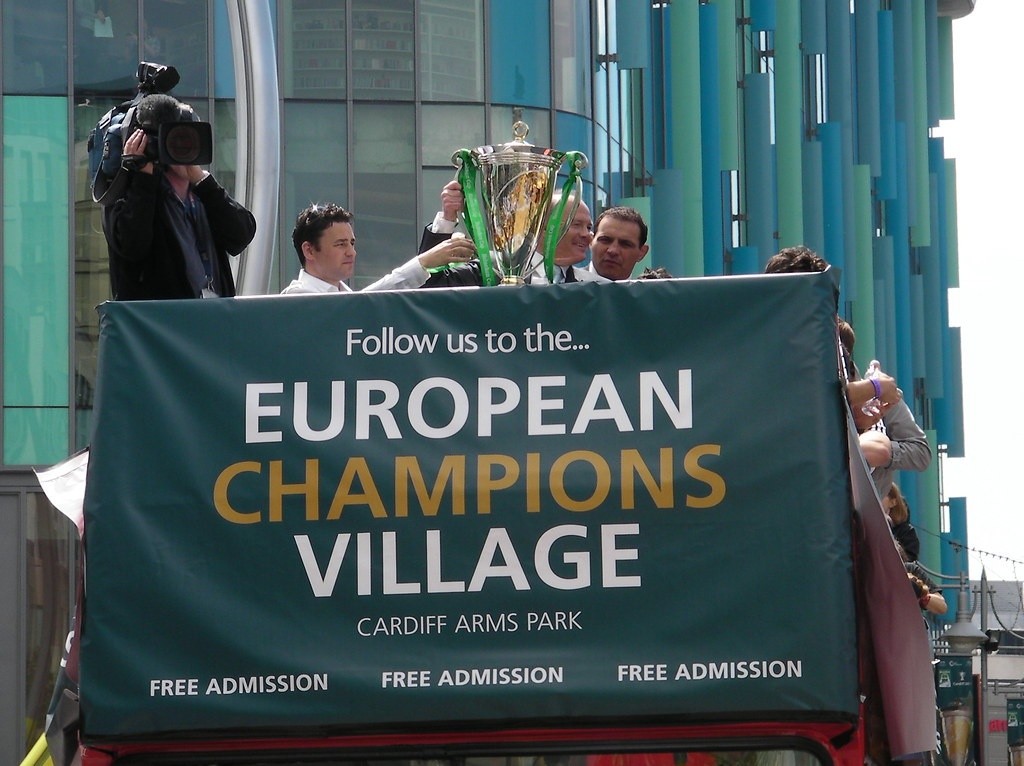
(869, 376), (881, 399)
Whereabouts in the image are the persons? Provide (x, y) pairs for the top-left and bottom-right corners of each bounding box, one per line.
(72, 0), (159, 107)
(766, 248), (949, 613)
(279, 203), (476, 295)
(420, 179), (674, 287)
(100, 96), (257, 301)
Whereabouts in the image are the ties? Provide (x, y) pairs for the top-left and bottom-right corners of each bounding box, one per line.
(553, 264), (564, 285)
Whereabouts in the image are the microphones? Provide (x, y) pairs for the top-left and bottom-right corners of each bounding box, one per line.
(133, 94), (182, 125)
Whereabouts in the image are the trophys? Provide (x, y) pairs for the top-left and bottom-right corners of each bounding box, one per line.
(453, 121), (586, 286)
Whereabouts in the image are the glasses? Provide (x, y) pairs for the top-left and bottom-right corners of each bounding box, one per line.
(306, 205), (328, 225)
